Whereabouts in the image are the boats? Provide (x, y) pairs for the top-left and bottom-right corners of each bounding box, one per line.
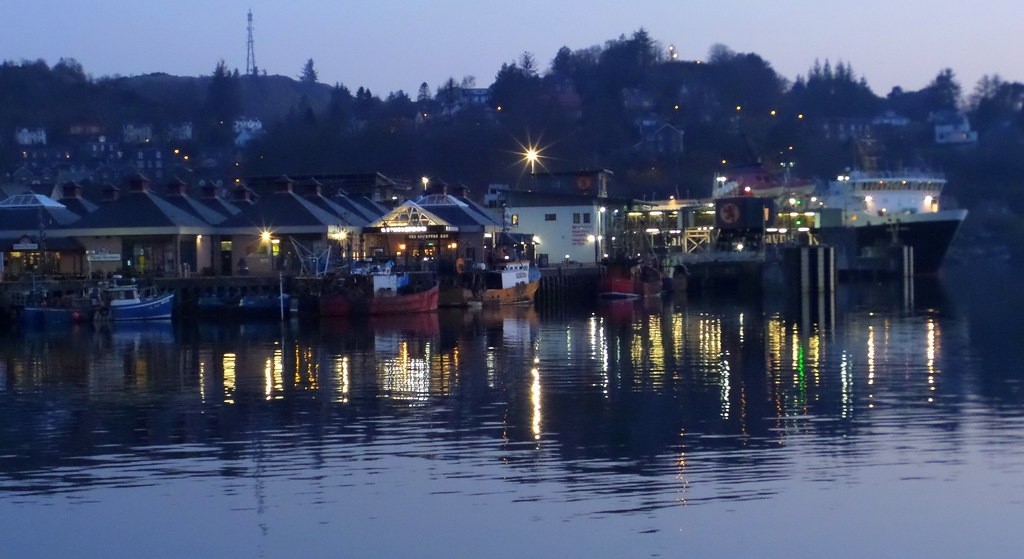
(20, 275), (176, 329)
(298, 280), (439, 315)
(193, 281), (291, 323)
(480, 281), (539, 305)
(596, 248), (644, 301)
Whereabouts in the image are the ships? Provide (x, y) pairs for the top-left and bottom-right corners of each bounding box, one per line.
(611, 173), (967, 284)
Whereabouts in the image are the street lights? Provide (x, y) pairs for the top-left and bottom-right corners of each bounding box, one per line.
(780, 162), (795, 192)
